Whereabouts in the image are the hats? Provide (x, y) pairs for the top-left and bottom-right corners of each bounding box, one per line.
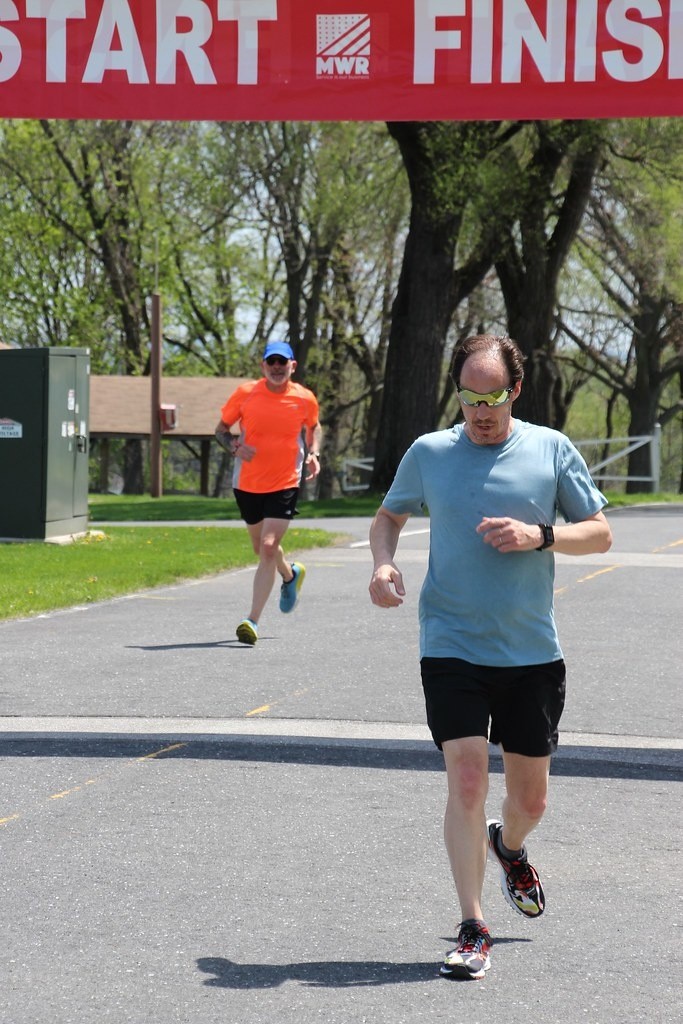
(263, 341), (294, 361)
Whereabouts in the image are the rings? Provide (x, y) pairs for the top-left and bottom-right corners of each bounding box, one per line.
(499, 536), (503, 543)
(498, 527), (504, 535)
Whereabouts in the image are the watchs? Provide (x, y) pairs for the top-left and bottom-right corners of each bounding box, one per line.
(535, 523), (555, 551)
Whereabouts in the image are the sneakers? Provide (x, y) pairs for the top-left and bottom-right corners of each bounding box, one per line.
(486, 819), (545, 918)
(439, 922), (493, 979)
(236, 619), (258, 645)
(280, 562), (306, 613)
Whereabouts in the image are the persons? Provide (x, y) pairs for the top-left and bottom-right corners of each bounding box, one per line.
(367, 333), (613, 981)
(215, 341), (322, 645)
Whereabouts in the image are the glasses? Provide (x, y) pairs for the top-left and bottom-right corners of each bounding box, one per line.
(266, 356), (288, 367)
(455, 378), (514, 407)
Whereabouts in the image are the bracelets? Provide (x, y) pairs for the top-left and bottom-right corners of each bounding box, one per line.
(308, 451), (320, 462)
(232, 444), (241, 457)
